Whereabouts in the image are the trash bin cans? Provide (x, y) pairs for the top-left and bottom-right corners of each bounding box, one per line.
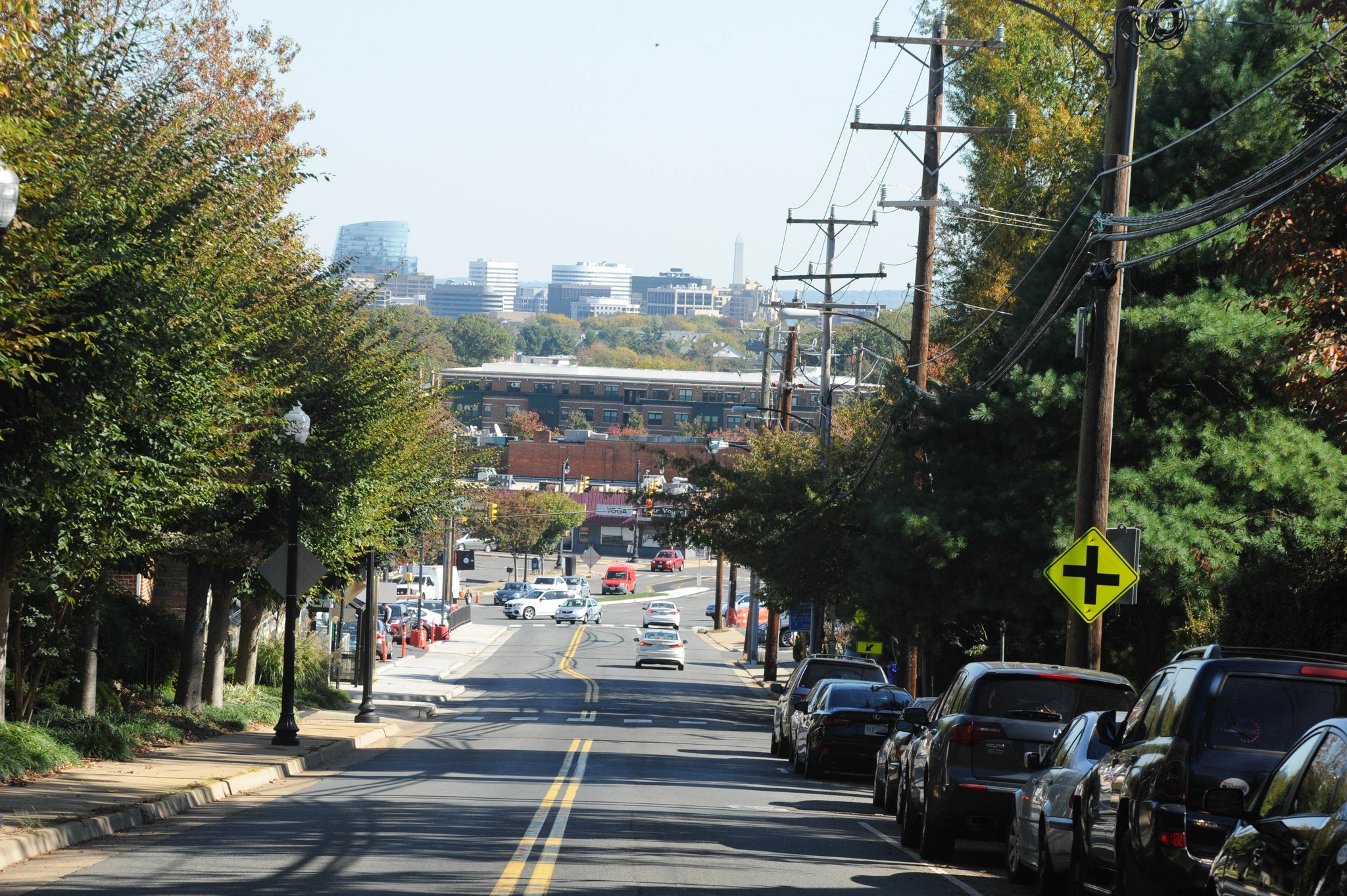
(564, 555), (576, 577)
(531, 558), (540, 570)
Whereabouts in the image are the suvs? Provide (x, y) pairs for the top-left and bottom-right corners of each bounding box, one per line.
(494, 575), (603, 625)
(650, 549), (685, 572)
(454, 530), (497, 552)
(705, 592), (768, 621)
(1067, 644), (1347, 895)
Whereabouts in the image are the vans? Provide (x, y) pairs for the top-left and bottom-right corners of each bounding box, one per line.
(601, 564), (637, 595)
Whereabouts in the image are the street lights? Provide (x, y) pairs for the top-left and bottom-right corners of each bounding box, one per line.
(270, 400), (312, 745)
(555, 458), (571, 569)
(630, 459), (664, 563)
(777, 305), (930, 700)
(729, 404), (831, 658)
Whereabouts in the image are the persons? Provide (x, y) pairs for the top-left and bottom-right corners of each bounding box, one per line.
(518, 585), (525, 592)
(550, 579), (555, 585)
(382, 603), (392, 633)
(576, 580), (582, 586)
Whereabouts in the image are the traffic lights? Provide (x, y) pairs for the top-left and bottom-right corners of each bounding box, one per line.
(577, 475), (590, 495)
(488, 503), (497, 522)
(629, 525), (635, 530)
(648, 478), (659, 496)
(458, 517), (467, 523)
(646, 499), (653, 517)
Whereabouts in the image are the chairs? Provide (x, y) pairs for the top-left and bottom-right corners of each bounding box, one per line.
(648, 634), (654, 639)
(577, 603), (581, 606)
(653, 604), (658, 607)
(348, 626), (355, 630)
(668, 635), (674, 640)
(508, 585), (513, 589)
(666, 605), (671, 608)
(571, 602), (576, 606)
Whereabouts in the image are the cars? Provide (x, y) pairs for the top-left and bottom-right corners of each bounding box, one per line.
(769, 641), (1128, 896)
(1202, 718), (1347, 896)
(757, 610), (792, 648)
(642, 601), (681, 630)
(302, 594), (458, 661)
(633, 629), (688, 671)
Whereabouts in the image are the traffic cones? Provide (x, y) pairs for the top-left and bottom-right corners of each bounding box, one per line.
(475, 589), (481, 603)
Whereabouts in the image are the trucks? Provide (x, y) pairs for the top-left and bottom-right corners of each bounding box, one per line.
(396, 565), (461, 604)
